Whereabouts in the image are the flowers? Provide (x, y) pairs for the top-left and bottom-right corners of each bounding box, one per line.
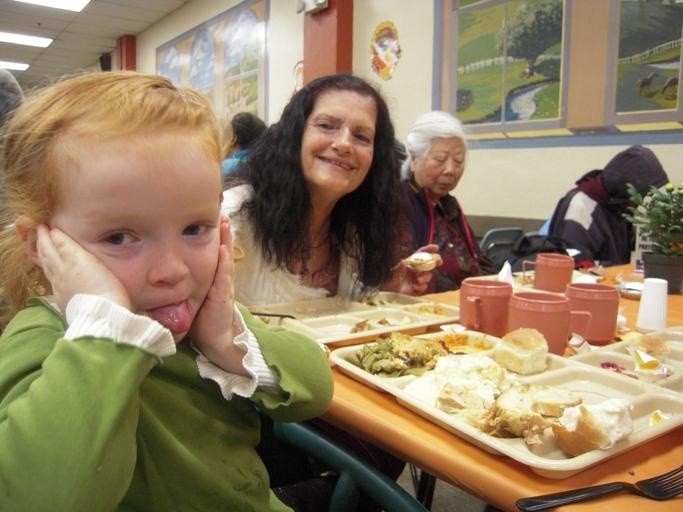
(623, 180), (683, 256)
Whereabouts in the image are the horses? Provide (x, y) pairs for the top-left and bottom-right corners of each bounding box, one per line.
(661, 76), (679, 95)
(635, 72), (660, 97)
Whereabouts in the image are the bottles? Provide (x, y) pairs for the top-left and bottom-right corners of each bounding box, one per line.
(635, 278), (669, 335)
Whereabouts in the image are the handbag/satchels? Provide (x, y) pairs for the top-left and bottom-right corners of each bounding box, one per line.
(486, 236), (569, 275)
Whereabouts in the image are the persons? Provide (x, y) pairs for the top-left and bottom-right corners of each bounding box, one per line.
(0, 69), (336, 512)
(218, 109), (271, 188)
(400, 111), (493, 298)
(538, 143), (670, 270)
(217, 73), (442, 312)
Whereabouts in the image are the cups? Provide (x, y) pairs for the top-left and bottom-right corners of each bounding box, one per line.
(458, 277), (513, 339)
(563, 282), (620, 346)
(522, 252), (576, 293)
(507, 289), (591, 357)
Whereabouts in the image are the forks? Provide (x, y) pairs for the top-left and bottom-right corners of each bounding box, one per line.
(515, 463), (682, 511)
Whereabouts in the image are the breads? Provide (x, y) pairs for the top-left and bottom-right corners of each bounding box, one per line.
(429, 328), (632, 457)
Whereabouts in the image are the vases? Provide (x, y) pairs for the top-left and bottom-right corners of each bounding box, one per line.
(642, 251), (683, 293)
